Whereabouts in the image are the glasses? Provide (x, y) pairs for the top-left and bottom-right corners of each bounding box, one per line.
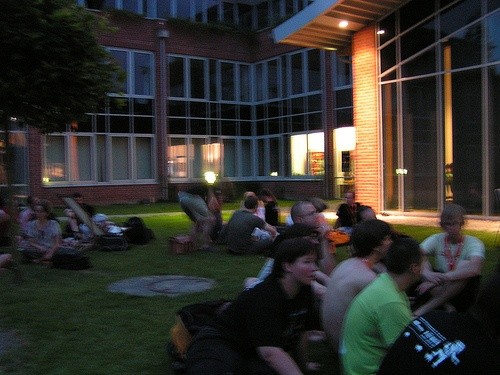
(300, 210), (317, 218)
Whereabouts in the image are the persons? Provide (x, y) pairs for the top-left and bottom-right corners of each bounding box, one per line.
(0, 193), (148, 269)
(175, 184), (500, 375)
